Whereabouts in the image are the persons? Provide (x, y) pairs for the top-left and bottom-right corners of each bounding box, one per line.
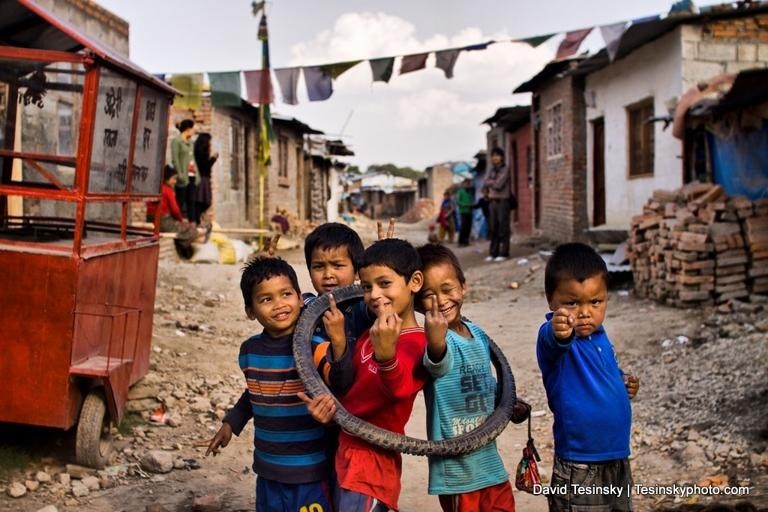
(446, 186), (463, 233)
(455, 178), (477, 246)
(147, 166), (213, 244)
(297, 239), (427, 511)
(171, 119), (200, 224)
(482, 146), (511, 262)
(193, 133), (220, 220)
(476, 186), (492, 238)
(435, 190), (457, 243)
(537, 243), (640, 511)
(412, 243), (531, 511)
(204, 256), (357, 512)
(302, 218), (395, 360)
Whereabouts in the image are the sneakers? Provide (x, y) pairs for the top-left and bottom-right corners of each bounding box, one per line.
(484, 257), (507, 263)
